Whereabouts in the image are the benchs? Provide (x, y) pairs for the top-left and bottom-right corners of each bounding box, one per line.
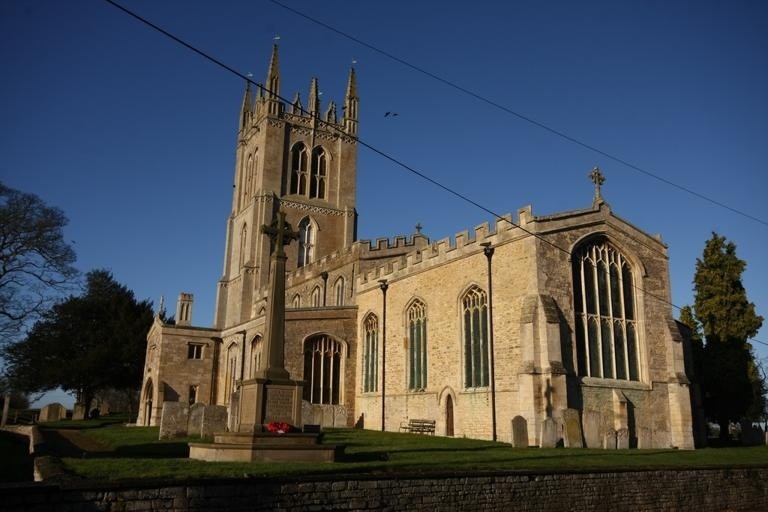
(399, 418), (436, 435)
